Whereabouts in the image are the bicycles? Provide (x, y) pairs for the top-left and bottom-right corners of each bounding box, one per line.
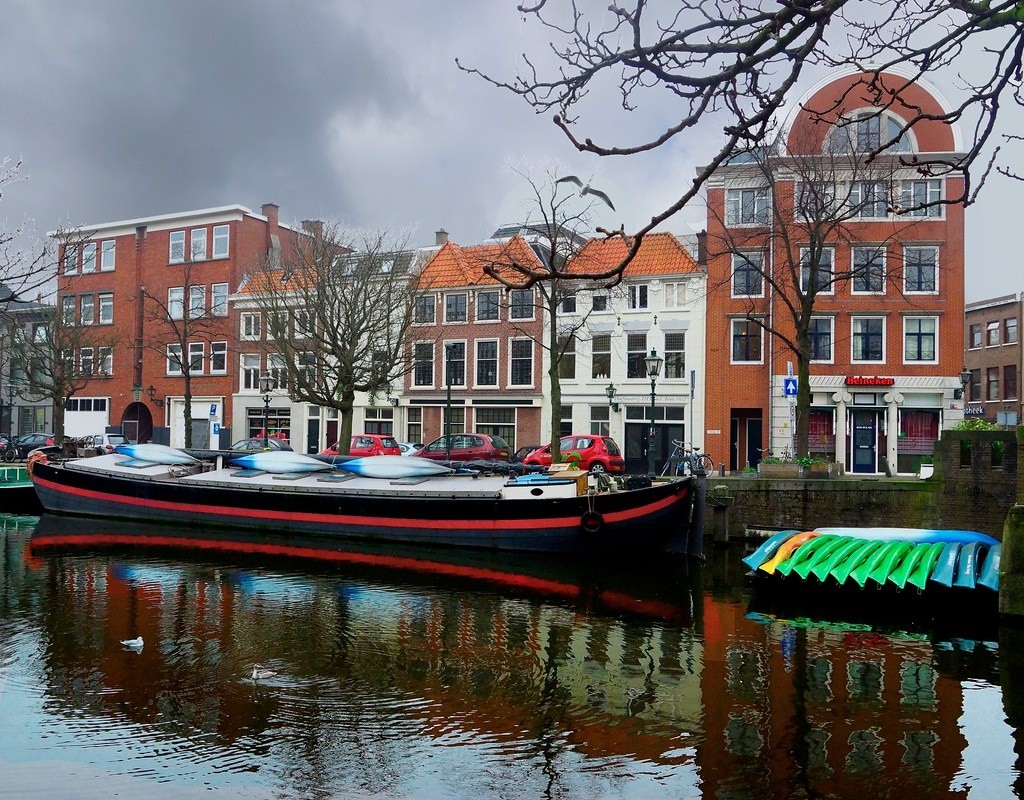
(660, 440), (715, 478)
(0, 445), (16, 462)
(757, 448), (778, 465)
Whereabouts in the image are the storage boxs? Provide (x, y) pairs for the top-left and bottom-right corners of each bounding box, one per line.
(549, 471), (588, 496)
(548, 463), (574, 475)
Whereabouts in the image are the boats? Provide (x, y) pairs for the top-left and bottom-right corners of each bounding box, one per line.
(28, 449), (696, 599)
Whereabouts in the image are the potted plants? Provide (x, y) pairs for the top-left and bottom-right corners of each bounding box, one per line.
(757, 455), (840, 479)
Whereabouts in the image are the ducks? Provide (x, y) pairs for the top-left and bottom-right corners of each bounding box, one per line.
(250, 663), (278, 680)
(118, 636), (144, 647)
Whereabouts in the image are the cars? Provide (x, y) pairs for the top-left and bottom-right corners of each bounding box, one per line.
(77, 433), (130, 458)
(227, 438), (294, 452)
(0, 433), (71, 459)
(522, 435), (624, 474)
(320, 434), (401, 457)
(409, 433), (511, 462)
(509, 446), (542, 463)
(385, 443), (424, 457)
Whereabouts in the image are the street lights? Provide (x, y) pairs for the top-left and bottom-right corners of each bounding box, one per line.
(445, 342), (456, 461)
(644, 348), (665, 479)
(259, 370), (276, 452)
(4, 380), (20, 462)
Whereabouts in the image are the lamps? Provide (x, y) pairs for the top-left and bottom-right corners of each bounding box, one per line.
(384, 382), (398, 407)
(953, 365), (973, 400)
(605, 382), (618, 412)
(146, 385), (163, 407)
